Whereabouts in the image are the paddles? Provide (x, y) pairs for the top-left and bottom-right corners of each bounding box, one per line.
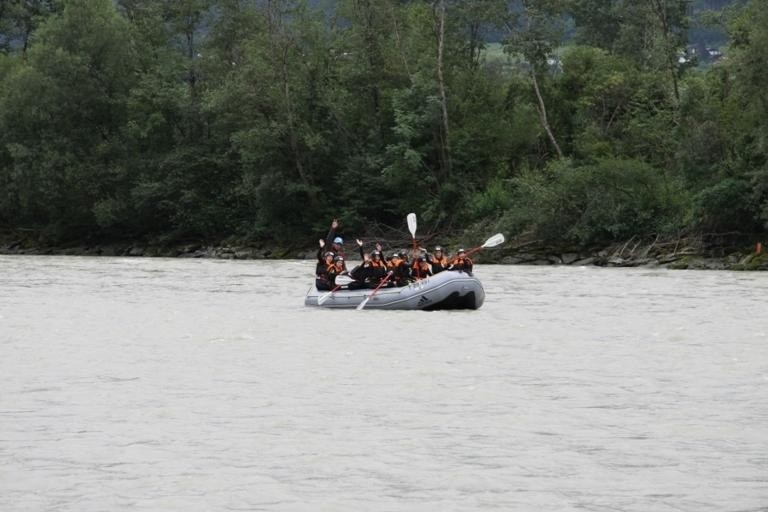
(354, 270), (394, 312)
(334, 275), (392, 287)
(317, 283), (340, 306)
(446, 233), (504, 264)
(407, 213), (420, 280)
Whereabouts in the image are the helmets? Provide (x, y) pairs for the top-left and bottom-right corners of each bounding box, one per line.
(325, 246), (466, 264)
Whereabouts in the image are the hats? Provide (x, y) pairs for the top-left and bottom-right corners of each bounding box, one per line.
(334, 237), (343, 245)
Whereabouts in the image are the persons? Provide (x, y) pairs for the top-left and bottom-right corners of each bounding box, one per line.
(313, 218), (474, 289)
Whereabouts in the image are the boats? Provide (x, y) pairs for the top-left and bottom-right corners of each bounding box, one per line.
(305, 270), (486, 310)
